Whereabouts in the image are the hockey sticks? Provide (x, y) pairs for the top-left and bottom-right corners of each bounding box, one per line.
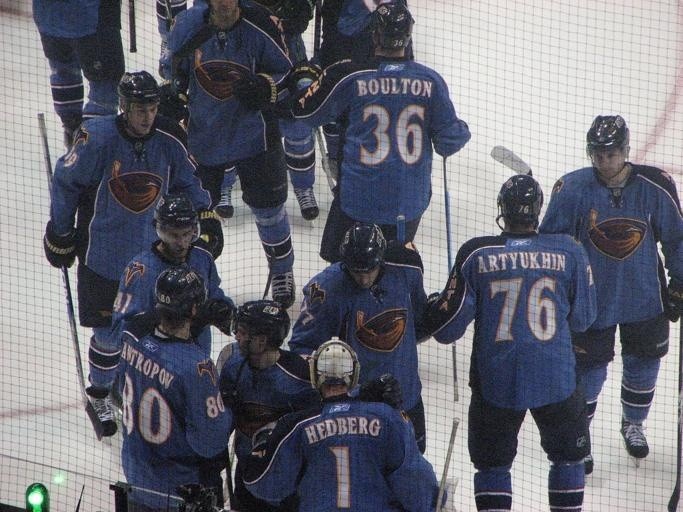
(489, 144), (533, 178)
(37, 113), (106, 442)
(668, 303), (681, 511)
(317, 126), (336, 196)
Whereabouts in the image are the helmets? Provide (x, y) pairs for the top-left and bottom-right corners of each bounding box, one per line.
(369, 2), (412, 47)
(496, 175), (542, 224)
(586, 115), (629, 157)
(117, 70), (161, 103)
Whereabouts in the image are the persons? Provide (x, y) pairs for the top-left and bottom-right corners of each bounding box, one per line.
(21, 1), (472, 512)
(429, 163), (603, 512)
(540, 108), (682, 481)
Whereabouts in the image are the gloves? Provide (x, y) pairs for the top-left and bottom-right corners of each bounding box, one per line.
(43, 221), (75, 268)
(666, 279), (682, 322)
(233, 74), (270, 110)
(286, 61), (322, 96)
(359, 374), (402, 409)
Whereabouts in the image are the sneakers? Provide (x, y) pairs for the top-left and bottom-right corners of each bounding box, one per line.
(621, 418), (648, 458)
(84, 387), (117, 436)
